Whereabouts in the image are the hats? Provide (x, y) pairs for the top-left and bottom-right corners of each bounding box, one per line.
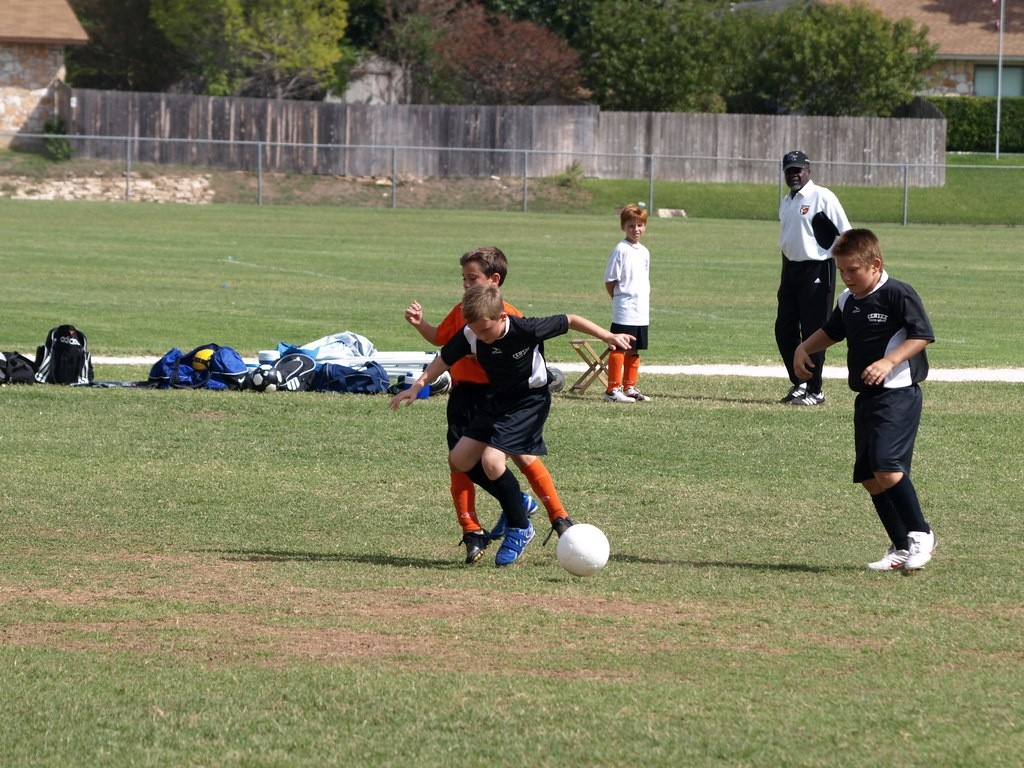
(782, 151), (811, 172)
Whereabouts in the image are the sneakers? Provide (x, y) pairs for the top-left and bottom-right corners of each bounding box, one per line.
(604, 387), (636, 403)
(495, 518), (535, 566)
(623, 385), (651, 402)
(787, 390), (826, 406)
(459, 528), (493, 564)
(904, 528), (938, 571)
(542, 516), (575, 547)
(867, 542), (910, 572)
(780, 386), (807, 405)
(489, 491), (539, 540)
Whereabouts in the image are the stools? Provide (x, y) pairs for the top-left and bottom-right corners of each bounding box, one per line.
(566, 338), (616, 395)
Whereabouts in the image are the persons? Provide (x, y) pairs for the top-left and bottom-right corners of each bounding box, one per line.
(793, 229), (937, 572)
(773, 151), (852, 405)
(404, 247), (573, 564)
(387, 283), (636, 566)
(604, 205), (653, 404)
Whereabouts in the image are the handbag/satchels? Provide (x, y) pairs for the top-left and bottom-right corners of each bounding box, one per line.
(146, 343), (250, 392)
(270, 349), (315, 394)
(0, 350), (39, 387)
(314, 358), (391, 395)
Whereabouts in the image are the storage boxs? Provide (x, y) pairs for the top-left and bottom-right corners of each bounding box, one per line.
(403, 371), (429, 399)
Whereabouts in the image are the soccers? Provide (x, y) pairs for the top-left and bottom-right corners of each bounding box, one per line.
(554, 522), (612, 579)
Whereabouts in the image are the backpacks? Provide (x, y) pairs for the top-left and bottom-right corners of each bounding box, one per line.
(35, 325), (96, 388)
